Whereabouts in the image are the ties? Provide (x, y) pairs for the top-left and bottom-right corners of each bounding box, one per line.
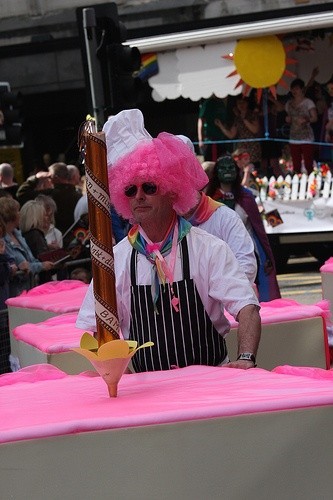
(187, 190), (225, 226)
(127, 214), (192, 316)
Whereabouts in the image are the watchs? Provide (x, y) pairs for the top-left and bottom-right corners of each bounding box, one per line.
(237, 353), (256, 365)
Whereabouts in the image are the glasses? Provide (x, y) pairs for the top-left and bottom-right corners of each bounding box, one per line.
(125, 181), (157, 196)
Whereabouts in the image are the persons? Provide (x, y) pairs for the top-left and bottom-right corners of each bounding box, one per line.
(208, 155), (281, 302)
(214, 93), (286, 177)
(76, 110), (262, 368)
(198, 95), (227, 161)
(0, 162), (87, 337)
(174, 135), (257, 291)
(232, 148), (258, 193)
(285, 67), (333, 174)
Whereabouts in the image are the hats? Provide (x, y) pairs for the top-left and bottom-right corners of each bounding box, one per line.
(104, 108), (154, 167)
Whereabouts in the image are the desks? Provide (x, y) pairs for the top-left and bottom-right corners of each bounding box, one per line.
(0, 364), (333, 499)
(5, 283), (91, 373)
(320, 260), (333, 335)
(14, 295), (329, 376)
